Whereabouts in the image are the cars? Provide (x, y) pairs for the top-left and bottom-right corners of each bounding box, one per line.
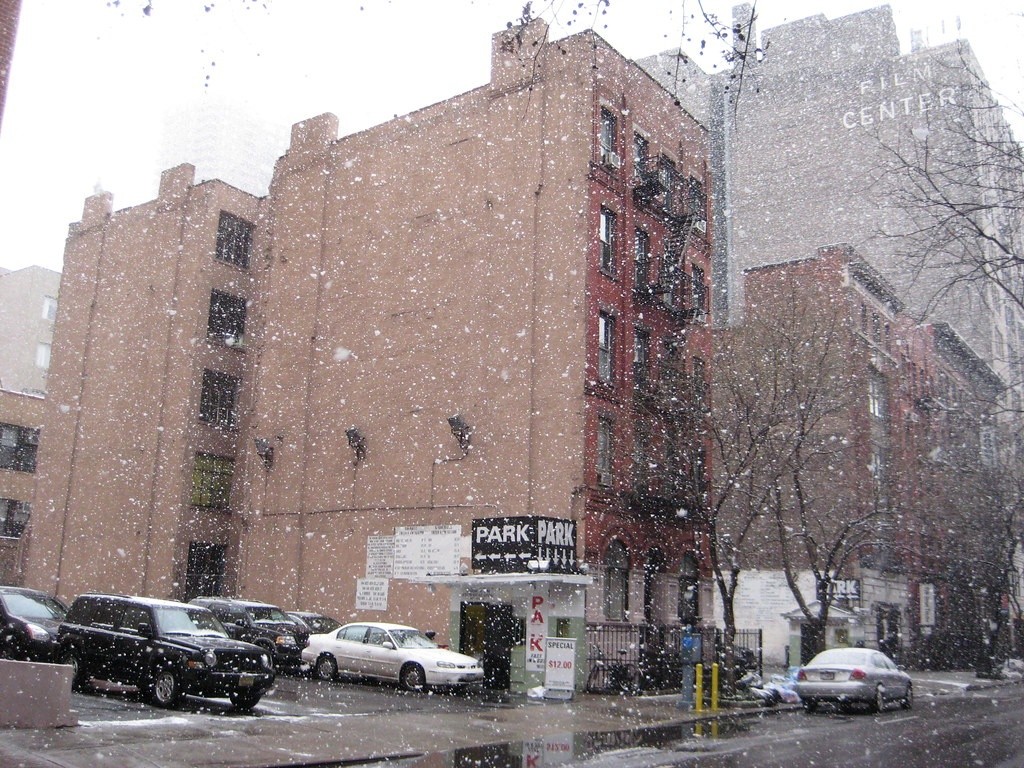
(284, 612), (343, 638)
(0, 588), (71, 667)
(797, 646), (914, 714)
(300, 621), (485, 693)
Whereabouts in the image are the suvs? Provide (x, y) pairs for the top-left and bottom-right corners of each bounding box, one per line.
(187, 595), (310, 675)
(50, 592), (276, 715)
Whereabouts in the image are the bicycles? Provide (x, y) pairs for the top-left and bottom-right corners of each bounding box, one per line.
(585, 647), (645, 695)
